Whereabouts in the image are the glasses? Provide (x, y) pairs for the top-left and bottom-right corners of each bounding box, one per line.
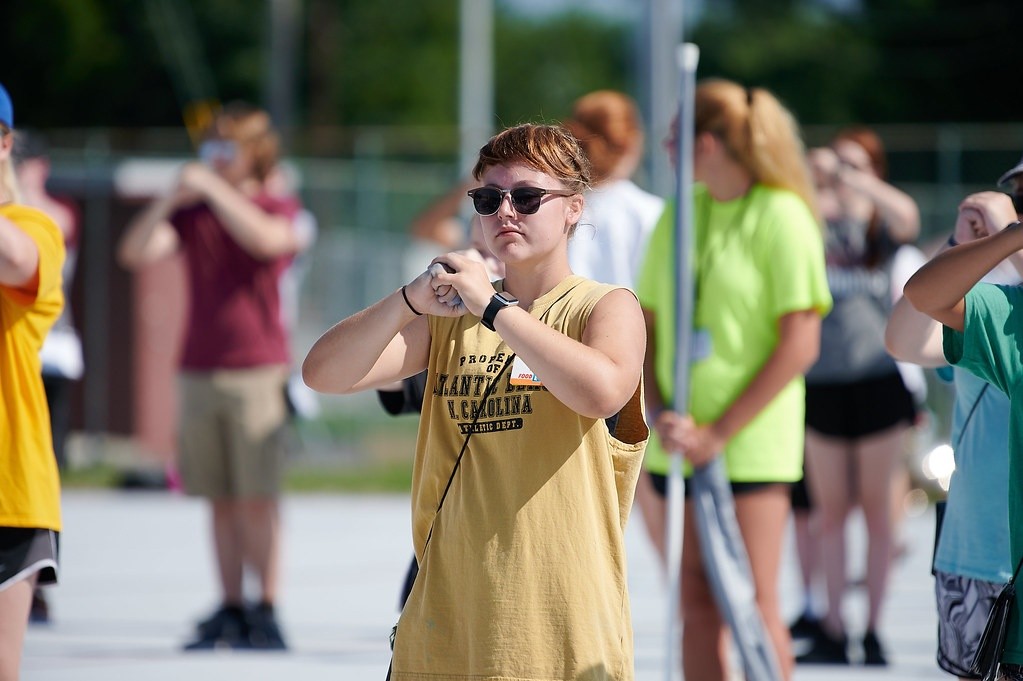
(467, 185), (577, 216)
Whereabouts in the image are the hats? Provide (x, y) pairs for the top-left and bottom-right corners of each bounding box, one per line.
(0, 81), (14, 131)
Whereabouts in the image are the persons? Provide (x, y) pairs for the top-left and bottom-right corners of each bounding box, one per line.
(886, 165), (1023, 681)
(791, 130), (927, 665)
(565, 91), (666, 559)
(638, 82), (834, 681)
(1, 87), (82, 681)
(904, 222), (1023, 681)
(376, 178), (505, 612)
(124, 109), (318, 650)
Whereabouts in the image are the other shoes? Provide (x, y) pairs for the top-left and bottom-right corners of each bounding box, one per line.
(796, 634), (848, 664)
(30, 594), (47, 622)
(862, 632), (885, 667)
(186, 598), (284, 650)
(790, 608), (822, 638)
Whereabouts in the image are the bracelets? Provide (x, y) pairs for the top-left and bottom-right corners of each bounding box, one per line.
(402, 285), (422, 316)
(948, 234), (959, 247)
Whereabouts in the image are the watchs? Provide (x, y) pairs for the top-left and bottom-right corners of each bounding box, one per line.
(481, 292), (519, 331)
